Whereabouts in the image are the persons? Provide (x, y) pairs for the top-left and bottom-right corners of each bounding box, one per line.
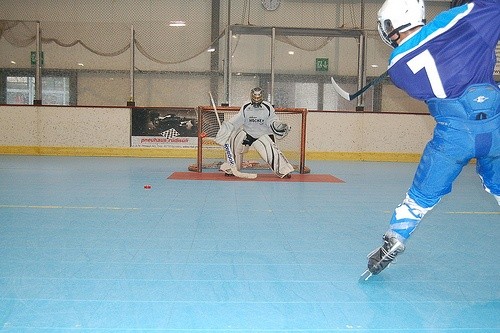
(358, 0), (500, 280)
(214, 87), (295, 179)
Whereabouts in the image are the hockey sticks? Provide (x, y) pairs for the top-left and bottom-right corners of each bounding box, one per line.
(331, 71), (391, 101)
(209, 91), (257, 179)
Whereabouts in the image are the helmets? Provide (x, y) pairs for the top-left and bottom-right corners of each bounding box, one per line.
(376, 0), (425, 48)
(250, 88), (263, 105)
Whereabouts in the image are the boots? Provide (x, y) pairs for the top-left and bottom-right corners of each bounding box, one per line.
(359, 234), (404, 280)
